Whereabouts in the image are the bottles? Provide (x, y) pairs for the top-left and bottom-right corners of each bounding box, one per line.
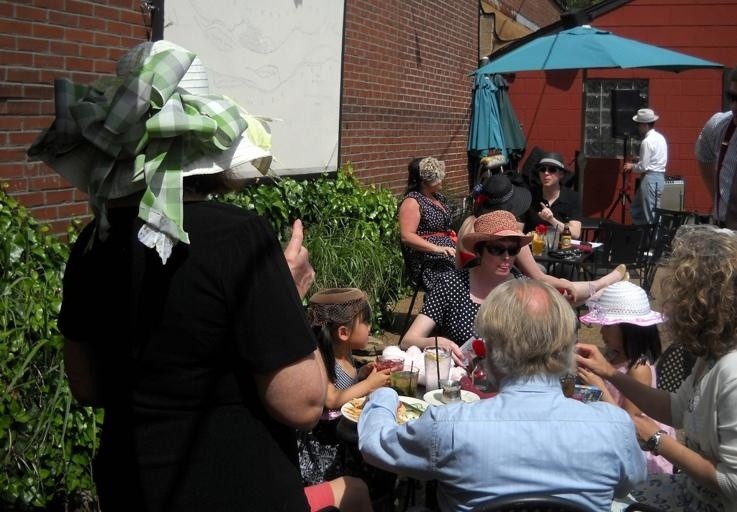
(560, 223), (570, 251)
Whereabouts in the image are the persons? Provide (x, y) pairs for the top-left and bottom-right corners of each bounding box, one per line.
(357, 277), (648, 512)
(520, 152), (582, 240)
(577, 282), (681, 474)
(295, 288), (404, 488)
(623, 108), (668, 265)
(26, 40), (328, 512)
(305, 476), (374, 512)
(456, 175), (630, 310)
(694, 65), (737, 230)
(397, 155), (458, 293)
(575, 224), (737, 512)
(401, 210), (533, 373)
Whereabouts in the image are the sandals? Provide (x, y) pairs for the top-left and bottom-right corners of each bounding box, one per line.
(622, 271), (630, 281)
(611, 262), (625, 280)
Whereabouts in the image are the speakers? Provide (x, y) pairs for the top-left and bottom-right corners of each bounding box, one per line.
(611, 89), (640, 138)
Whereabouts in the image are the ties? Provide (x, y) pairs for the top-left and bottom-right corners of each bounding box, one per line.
(722, 164), (736, 229)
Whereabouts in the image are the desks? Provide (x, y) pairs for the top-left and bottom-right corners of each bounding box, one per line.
(515, 241), (602, 284)
(566, 218), (616, 241)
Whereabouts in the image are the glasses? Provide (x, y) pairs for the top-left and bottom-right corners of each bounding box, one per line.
(537, 166), (559, 173)
(484, 243), (521, 256)
(725, 91), (737, 99)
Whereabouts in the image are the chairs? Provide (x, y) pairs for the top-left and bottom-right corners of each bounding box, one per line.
(469, 494), (593, 511)
(398, 240), (450, 346)
(583, 208), (716, 311)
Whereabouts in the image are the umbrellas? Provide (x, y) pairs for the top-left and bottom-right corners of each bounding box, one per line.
(491, 74), (527, 154)
(468, 73), (507, 178)
(468, 25), (726, 217)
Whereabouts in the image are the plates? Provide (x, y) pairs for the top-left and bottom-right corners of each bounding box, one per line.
(341, 388), (480, 426)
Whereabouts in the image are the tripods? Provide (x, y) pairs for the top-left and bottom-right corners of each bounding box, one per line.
(593, 141), (632, 242)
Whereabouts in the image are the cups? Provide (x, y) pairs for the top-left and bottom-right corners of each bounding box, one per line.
(547, 226), (559, 251)
(375, 345), (578, 405)
(531, 230), (545, 255)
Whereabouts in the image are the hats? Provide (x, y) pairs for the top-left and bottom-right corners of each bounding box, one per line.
(462, 210), (535, 254)
(309, 287), (365, 305)
(632, 108), (660, 124)
(472, 173), (533, 218)
(407, 155), (432, 183)
(579, 280), (666, 328)
(535, 151), (565, 170)
(94, 40), (274, 199)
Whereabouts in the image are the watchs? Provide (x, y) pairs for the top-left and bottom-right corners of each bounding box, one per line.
(647, 429), (668, 456)
